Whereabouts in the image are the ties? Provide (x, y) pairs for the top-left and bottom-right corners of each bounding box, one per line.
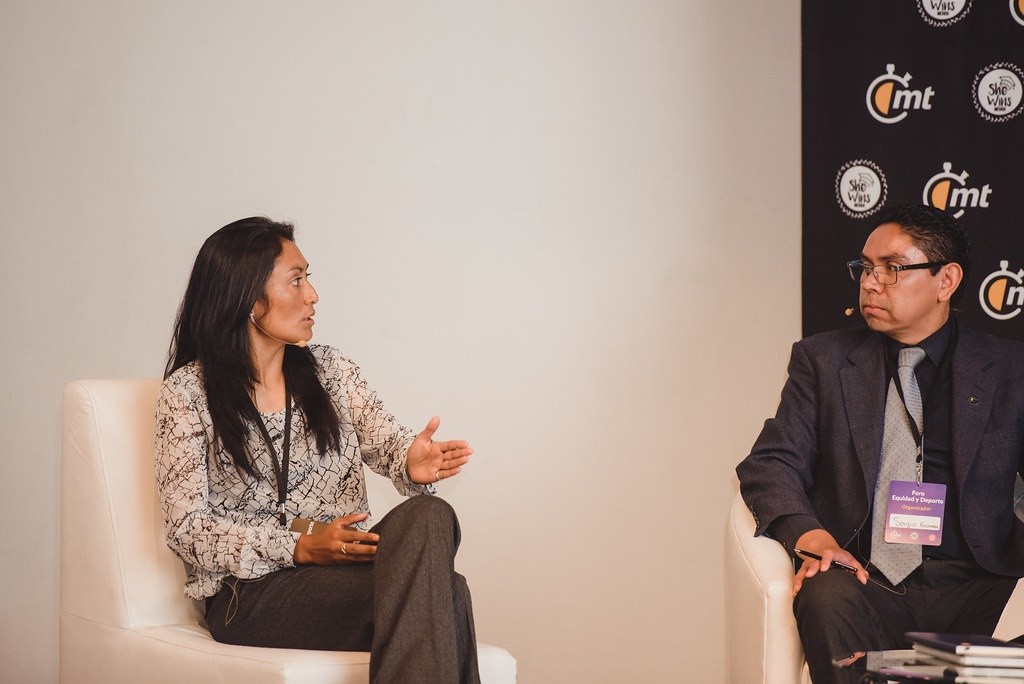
(871, 345), (924, 587)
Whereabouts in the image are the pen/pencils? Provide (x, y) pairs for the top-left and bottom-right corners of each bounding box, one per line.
(793, 547), (858, 573)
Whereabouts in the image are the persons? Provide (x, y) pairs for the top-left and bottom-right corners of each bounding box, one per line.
(155, 216), (481, 684)
(735, 205), (1024, 684)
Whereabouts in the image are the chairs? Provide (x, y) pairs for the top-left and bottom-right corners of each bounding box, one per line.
(59, 379), (520, 684)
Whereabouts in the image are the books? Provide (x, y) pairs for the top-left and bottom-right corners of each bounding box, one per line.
(902, 632), (1024, 684)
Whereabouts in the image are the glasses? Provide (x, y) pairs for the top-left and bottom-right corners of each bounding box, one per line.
(848, 257), (944, 286)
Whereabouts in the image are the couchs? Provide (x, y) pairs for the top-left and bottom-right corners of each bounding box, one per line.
(726, 474), (1024, 684)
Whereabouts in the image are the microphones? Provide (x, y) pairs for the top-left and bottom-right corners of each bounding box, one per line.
(845, 304), (859, 316)
(249, 314), (306, 347)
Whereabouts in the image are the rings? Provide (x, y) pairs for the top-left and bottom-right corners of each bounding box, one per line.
(436, 472), (440, 480)
(341, 544), (347, 555)
